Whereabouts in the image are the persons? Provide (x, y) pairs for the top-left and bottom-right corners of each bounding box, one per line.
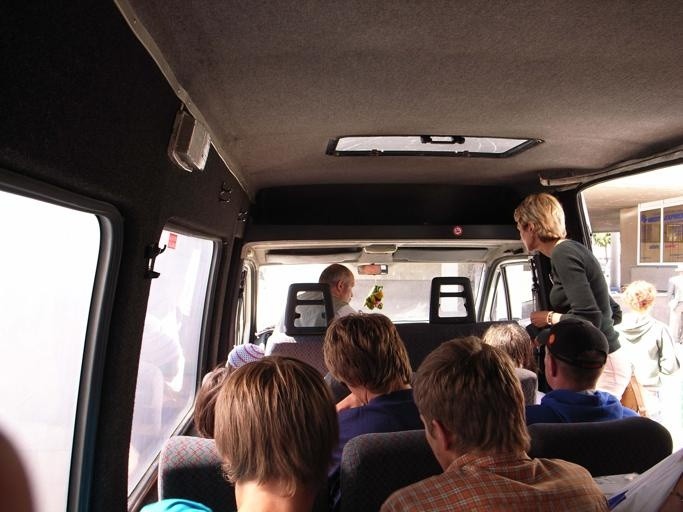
(510, 192), (633, 404)
(139, 263), (681, 512)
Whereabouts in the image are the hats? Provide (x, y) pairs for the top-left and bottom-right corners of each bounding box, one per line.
(535, 317), (607, 365)
(227, 343), (264, 368)
